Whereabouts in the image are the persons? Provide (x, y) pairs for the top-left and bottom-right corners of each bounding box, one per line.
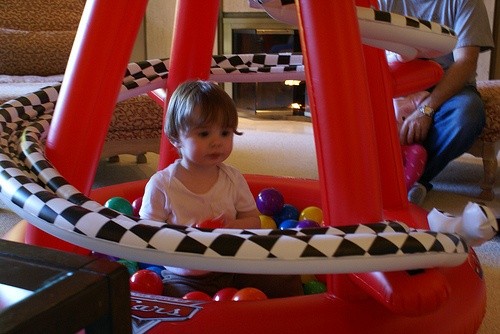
(256, 0), (494, 209)
(138, 79), (305, 300)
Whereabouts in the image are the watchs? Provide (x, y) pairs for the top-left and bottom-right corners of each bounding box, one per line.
(417, 105), (435, 118)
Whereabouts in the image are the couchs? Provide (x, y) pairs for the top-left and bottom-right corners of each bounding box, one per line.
(0, 0), (168, 166)
